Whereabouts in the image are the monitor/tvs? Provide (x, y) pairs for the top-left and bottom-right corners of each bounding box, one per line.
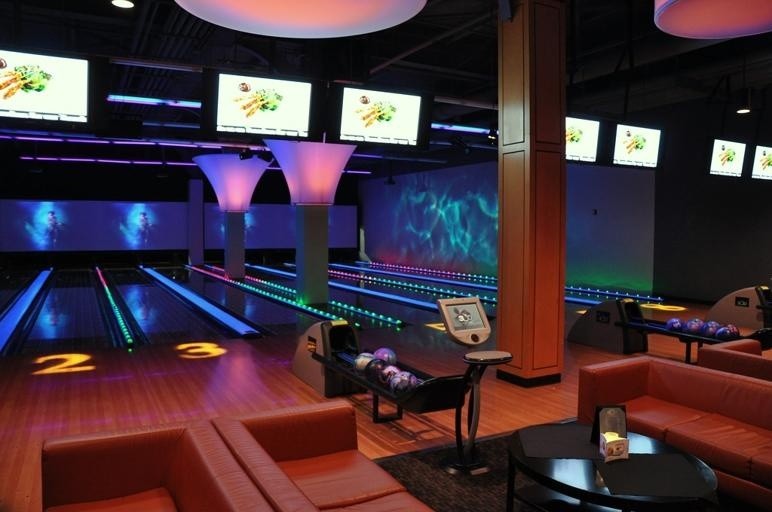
(437, 297), (491, 346)
(751, 145), (772, 182)
(612, 123), (662, 169)
(564, 115), (604, 164)
(0, 45), (93, 134)
(709, 139), (746, 178)
(207, 70), (314, 140)
(325, 83), (434, 151)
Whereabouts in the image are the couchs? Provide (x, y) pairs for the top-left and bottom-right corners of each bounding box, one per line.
(209, 399), (436, 511)
(40, 417), (275, 511)
(576, 337), (771, 508)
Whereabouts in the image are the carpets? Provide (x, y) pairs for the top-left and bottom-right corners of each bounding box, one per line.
(370, 417), (575, 511)
